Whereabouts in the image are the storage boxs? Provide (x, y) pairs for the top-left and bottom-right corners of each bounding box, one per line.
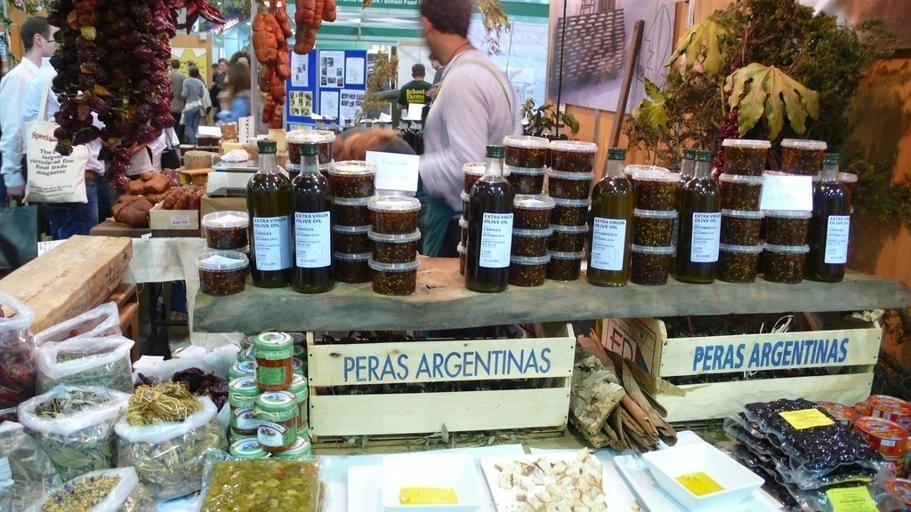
(200, 194), (248, 241)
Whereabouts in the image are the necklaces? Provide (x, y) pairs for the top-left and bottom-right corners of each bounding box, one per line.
(447, 41), (471, 64)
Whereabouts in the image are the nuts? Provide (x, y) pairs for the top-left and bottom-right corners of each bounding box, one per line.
(493, 446), (609, 512)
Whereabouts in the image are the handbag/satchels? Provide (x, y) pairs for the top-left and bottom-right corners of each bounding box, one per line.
(21, 86), (90, 206)
(0, 204), (40, 271)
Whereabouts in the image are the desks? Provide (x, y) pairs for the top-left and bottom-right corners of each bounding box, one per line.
(91, 220), (204, 343)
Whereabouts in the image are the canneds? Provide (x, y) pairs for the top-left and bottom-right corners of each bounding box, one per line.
(816, 391), (911, 511)
(290, 357), (304, 375)
(286, 331), (308, 346)
(226, 430), (255, 443)
(236, 348), (256, 365)
(240, 334), (257, 349)
(254, 331), (294, 391)
(272, 435), (314, 467)
(228, 437), (273, 462)
(256, 390), (298, 452)
(284, 372), (309, 436)
(289, 342), (303, 355)
(228, 375), (263, 436)
(226, 358), (256, 382)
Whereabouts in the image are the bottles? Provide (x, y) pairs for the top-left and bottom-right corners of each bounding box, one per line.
(669, 149), (699, 276)
(291, 140), (336, 293)
(464, 144), (514, 293)
(586, 147), (636, 288)
(673, 150), (722, 285)
(248, 136), (294, 288)
(807, 153), (853, 282)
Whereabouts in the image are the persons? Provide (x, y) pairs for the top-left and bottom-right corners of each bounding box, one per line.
(180, 51), (252, 144)
(0, 16), (181, 239)
(335, 49), (447, 158)
(413, 0), (524, 341)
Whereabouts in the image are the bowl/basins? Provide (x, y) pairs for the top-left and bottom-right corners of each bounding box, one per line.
(502, 133), (599, 287)
(368, 193), (424, 296)
(623, 163), (679, 286)
(456, 160), (513, 276)
(327, 157), (377, 282)
(717, 136), (829, 282)
(195, 209), (250, 296)
(813, 171), (859, 207)
(178, 135), (222, 166)
(285, 129), (336, 181)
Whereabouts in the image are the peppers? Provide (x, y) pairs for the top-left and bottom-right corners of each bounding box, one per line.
(49, 0), (179, 196)
(171, 0), (229, 38)
